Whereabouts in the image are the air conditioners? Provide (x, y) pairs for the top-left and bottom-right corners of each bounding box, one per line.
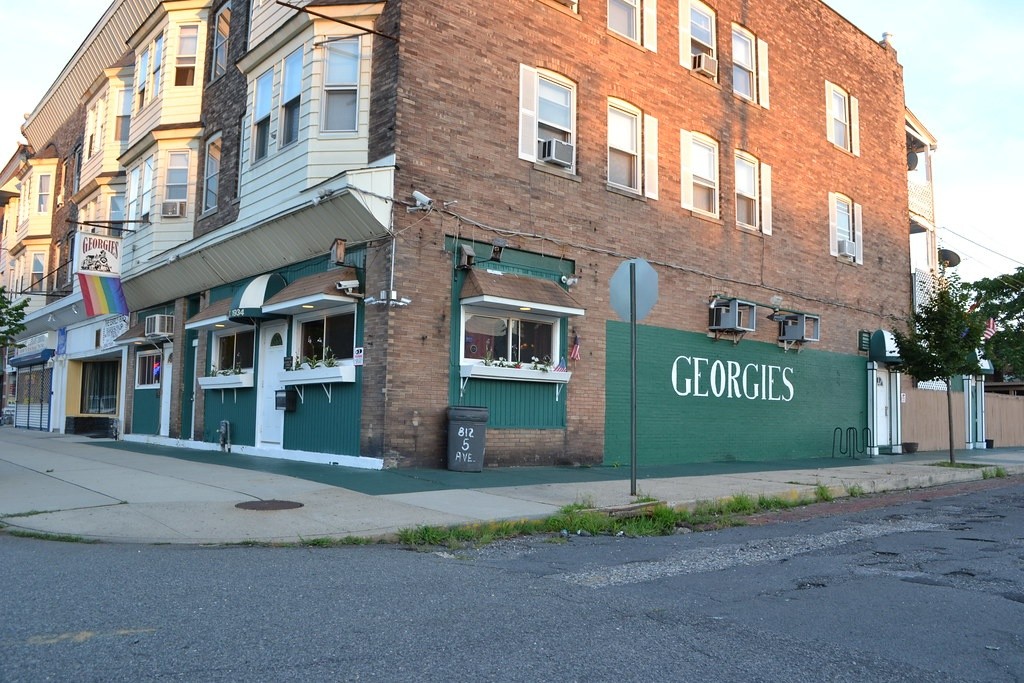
(780, 320), (803, 336)
(838, 241), (856, 257)
(144, 314), (174, 337)
(694, 53), (717, 78)
(545, 139), (574, 166)
(161, 200), (183, 217)
(710, 307), (743, 326)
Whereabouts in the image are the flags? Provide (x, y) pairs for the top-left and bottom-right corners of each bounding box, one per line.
(571, 336), (580, 360)
(78, 274), (129, 317)
(554, 358), (566, 372)
(984, 317), (996, 339)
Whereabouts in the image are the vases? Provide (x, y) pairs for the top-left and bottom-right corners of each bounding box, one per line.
(903, 442), (918, 453)
(461, 364), (573, 383)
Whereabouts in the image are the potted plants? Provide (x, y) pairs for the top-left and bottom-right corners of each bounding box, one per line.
(275, 347), (356, 386)
(196, 352), (254, 390)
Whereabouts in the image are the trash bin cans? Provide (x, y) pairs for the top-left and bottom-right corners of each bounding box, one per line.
(444, 402), (489, 475)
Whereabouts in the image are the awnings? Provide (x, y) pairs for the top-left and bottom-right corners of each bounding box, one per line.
(870, 330), (904, 363)
(975, 348), (994, 374)
(229, 273), (288, 325)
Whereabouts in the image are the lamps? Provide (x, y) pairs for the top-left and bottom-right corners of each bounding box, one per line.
(47, 355), (53, 368)
(72, 304), (80, 315)
(454, 237), (507, 271)
(48, 314), (56, 322)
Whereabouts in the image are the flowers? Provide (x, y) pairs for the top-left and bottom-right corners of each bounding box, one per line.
(481, 352), (556, 373)
